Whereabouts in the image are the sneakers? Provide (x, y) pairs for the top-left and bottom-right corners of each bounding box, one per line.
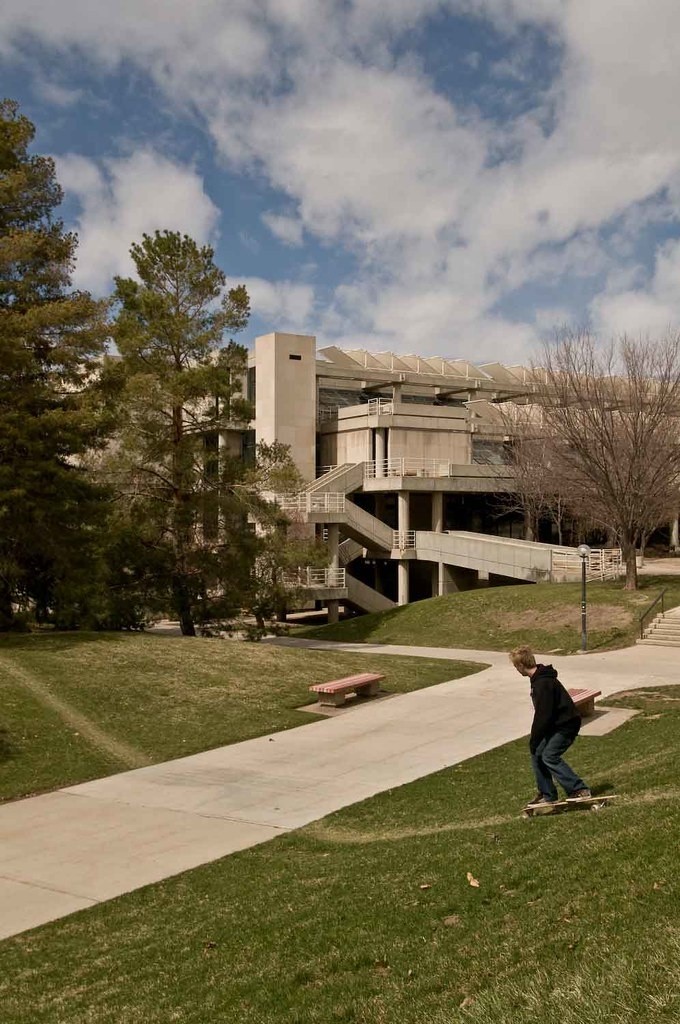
(566, 789), (592, 801)
(527, 795), (558, 808)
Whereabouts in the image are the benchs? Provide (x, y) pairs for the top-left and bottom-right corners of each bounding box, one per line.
(309, 672), (386, 707)
(567, 688), (601, 716)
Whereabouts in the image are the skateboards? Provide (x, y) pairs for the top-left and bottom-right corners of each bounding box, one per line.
(522, 793), (616, 814)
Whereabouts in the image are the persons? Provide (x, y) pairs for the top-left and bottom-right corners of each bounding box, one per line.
(509, 643), (593, 808)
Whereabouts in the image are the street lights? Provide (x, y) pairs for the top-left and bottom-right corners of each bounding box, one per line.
(577, 543), (592, 652)
